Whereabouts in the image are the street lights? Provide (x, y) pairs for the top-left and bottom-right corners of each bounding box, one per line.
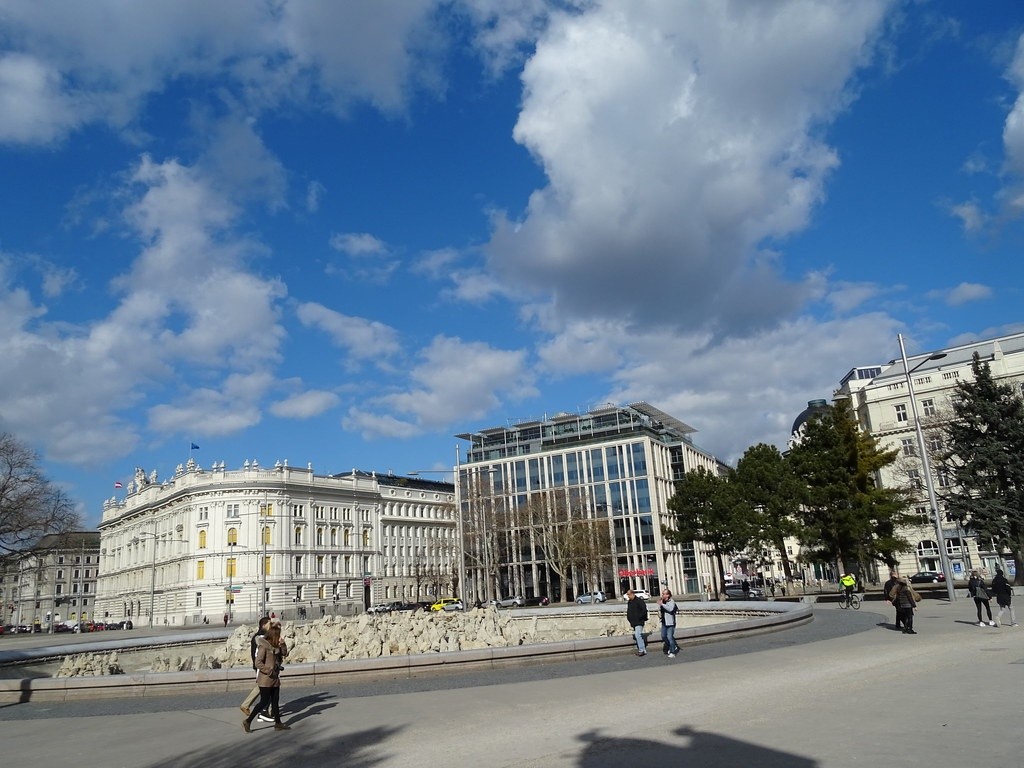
(140, 520), (188, 627)
(229, 501), (304, 619)
(406, 444), (499, 612)
(887, 331), (958, 603)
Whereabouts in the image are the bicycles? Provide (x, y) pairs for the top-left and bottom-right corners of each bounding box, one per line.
(838, 589), (861, 610)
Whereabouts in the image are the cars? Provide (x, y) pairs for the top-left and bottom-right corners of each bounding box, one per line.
(481, 600), (497, 609)
(0, 621), (133, 635)
(622, 590), (652, 603)
(366, 601), (432, 613)
(525, 596), (550, 606)
(908, 572), (945, 583)
(441, 600), (463, 611)
(496, 594), (526, 609)
(720, 584), (762, 597)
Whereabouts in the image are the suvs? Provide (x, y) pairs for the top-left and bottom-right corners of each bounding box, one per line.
(575, 591), (607, 604)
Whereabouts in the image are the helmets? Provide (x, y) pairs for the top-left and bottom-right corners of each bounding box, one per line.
(840, 574), (845, 578)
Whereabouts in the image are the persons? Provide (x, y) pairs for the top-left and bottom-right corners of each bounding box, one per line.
(781, 587), (785, 596)
(968, 571), (995, 627)
(992, 570), (1019, 627)
(473, 599), (481, 608)
(995, 563), (1000, 572)
(271, 612), (275, 618)
(266, 612), (269, 617)
(884, 571), (917, 634)
(771, 584), (774, 596)
(239, 617), (291, 731)
(837, 574), (854, 601)
(657, 589), (678, 658)
(627, 590), (648, 655)
(223, 613), (228, 627)
(202, 615), (208, 624)
(743, 579), (750, 597)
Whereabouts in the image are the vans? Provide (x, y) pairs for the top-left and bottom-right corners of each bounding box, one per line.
(430, 597), (461, 613)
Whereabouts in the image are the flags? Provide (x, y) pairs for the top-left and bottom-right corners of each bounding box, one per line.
(191, 443), (199, 449)
(115, 483), (122, 488)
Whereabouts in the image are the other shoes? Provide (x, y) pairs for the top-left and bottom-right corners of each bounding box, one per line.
(995, 619), (1001, 627)
(979, 622), (986, 626)
(257, 714), (264, 721)
(243, 719), (253, 733)
(989, 621), (995, 625)
(668, 647), (677, 654)
(259, 711), (275, 721)
(636, 652), (644, 656)
(907, 629), (917, 634)
(240, 705), (250, 716)
(669, 653), (675, 658)
(274, 724), (290, 731)
(1012, 623), (1018, 626)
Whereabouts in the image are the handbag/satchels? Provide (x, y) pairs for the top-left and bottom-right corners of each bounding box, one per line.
(975, 581), (989, 599)
